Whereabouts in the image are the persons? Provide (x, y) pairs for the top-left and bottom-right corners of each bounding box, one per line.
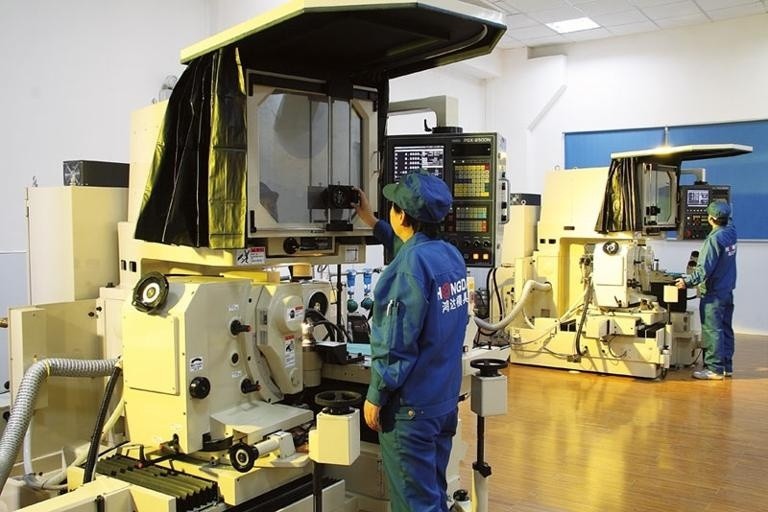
(675, 199), (738, 381)
(258, 184), (279, 223)
(349, 171), (470, 511)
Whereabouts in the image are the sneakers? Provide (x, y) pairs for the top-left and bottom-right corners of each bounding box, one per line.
(693, 368), (733, 379)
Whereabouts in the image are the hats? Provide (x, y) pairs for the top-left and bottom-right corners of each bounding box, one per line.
(382, 168), (453, 223)
(706, 202), (731, 220)
